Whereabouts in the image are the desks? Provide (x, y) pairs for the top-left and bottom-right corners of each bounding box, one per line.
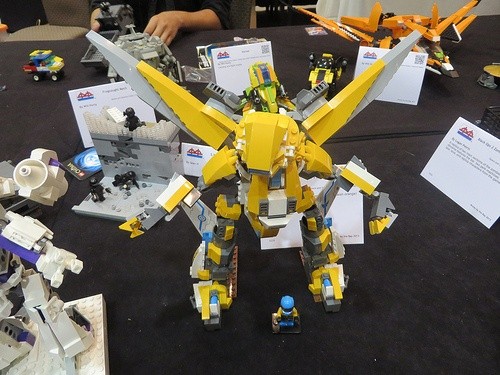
(0, 12), (500, 374)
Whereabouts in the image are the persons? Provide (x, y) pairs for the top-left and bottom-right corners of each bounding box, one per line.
(87, 25), (423, 330)
(277, 295), (299, 329)
(0, 148), (97, 373)
(88, 0), (231, 45)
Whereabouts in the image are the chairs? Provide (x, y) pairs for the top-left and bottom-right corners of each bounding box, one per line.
(4, 1), (92, 44)
